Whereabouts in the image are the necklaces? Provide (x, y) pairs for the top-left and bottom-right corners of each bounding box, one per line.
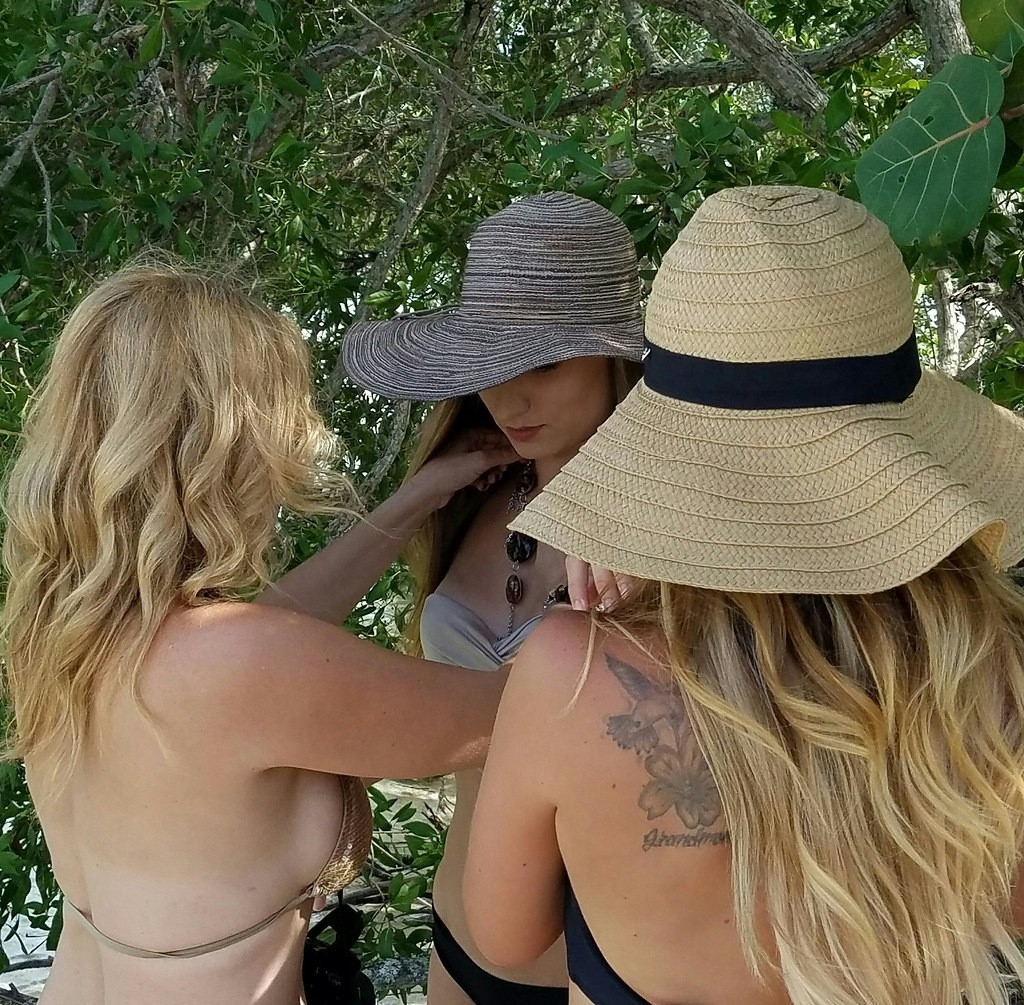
(504, 459), (577, 636)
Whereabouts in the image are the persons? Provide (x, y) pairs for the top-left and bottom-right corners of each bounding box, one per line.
(343, 191), (651, 1005)
(462, 185), (1024, 1005)
(0, 249), (522, 1005)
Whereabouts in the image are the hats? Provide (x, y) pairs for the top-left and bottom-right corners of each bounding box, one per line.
(508, 184), (1023, 595)
(340, 191), (645, 399)
